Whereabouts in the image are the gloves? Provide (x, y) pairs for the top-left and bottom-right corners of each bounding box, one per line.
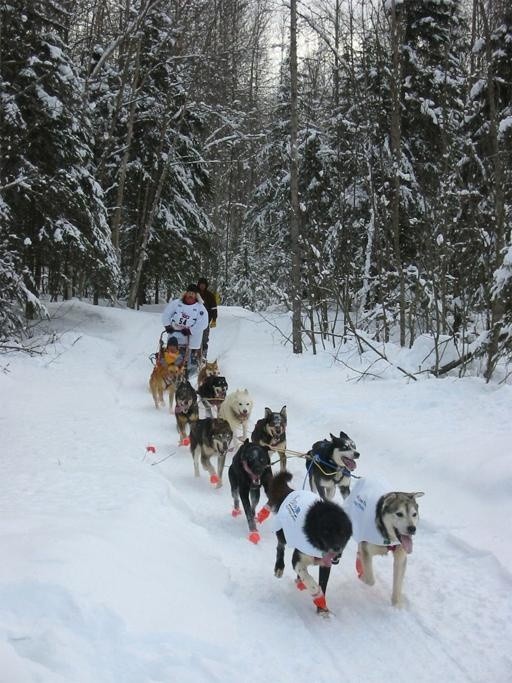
(210, 320), (216, 328)
(181, 328), (191, 336)
(165, 325), (174, 334)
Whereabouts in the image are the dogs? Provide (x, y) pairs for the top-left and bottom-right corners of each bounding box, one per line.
(150, 359), (227, 447)
(217, 389), (254, 453)
(251, 405), (287, 474)
(228, 438), (273, 545)
(189, 417), (232, 489)
(268, 471), (352, 623)
(306, 432), (360, 502)
(344, 492), (425, 607)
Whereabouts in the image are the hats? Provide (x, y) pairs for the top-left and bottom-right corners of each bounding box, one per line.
(167, 336), (178, 350)
(187, 278), (209, 293)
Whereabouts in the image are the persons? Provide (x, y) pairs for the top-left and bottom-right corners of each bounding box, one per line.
(160, 278), (217, 381)
